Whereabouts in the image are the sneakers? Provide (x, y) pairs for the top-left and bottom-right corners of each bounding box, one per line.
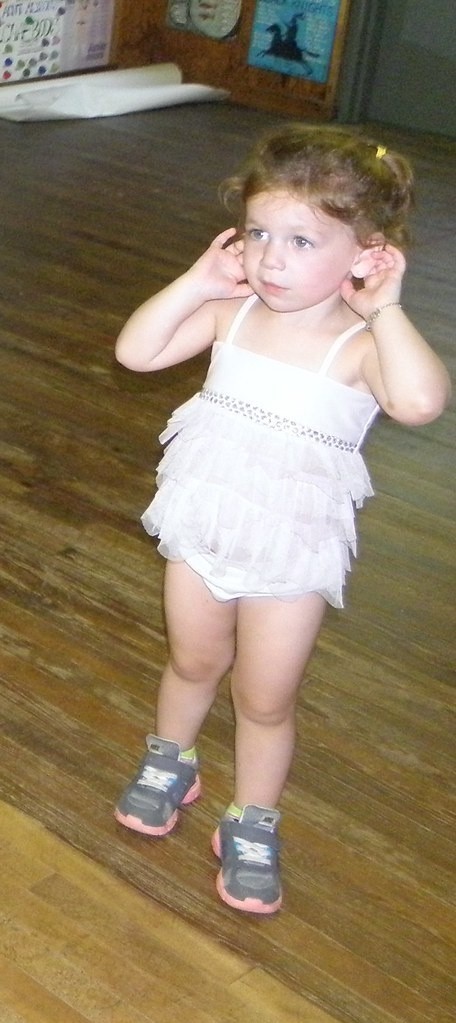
(211, 804), (283, 914)
(113, 733), (201, 836)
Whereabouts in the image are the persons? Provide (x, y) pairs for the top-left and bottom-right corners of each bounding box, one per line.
(113, 126), (452, 914)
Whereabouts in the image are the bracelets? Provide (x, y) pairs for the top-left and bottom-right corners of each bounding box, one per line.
(366, 302), (401, 331)
